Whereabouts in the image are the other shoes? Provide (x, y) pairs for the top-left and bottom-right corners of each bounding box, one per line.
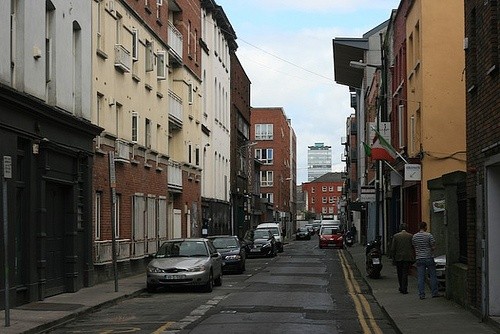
(420, 296), (425, 298)
(432, 292), (444, 297)
(400, 289), (408, 294)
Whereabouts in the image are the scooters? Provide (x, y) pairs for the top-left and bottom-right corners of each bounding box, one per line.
(345, 234), (353, 247)
(364, 236), (382, 279)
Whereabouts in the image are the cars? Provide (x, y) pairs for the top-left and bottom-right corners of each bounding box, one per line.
(145, 235), (250, 293)
(426, 254), (446, 283)
(296, 220), (344, 247)
(248, 230), (277, 258)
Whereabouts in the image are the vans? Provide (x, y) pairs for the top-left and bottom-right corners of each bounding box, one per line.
(256, 223), (284, 253)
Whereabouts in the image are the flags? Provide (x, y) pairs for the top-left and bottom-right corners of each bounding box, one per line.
(363, 131), (395, 164)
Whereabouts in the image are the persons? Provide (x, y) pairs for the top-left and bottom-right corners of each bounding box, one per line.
(390, 222), (416, 294)
(350, 224), (357, 243)
(412, 221), (439, 299)
